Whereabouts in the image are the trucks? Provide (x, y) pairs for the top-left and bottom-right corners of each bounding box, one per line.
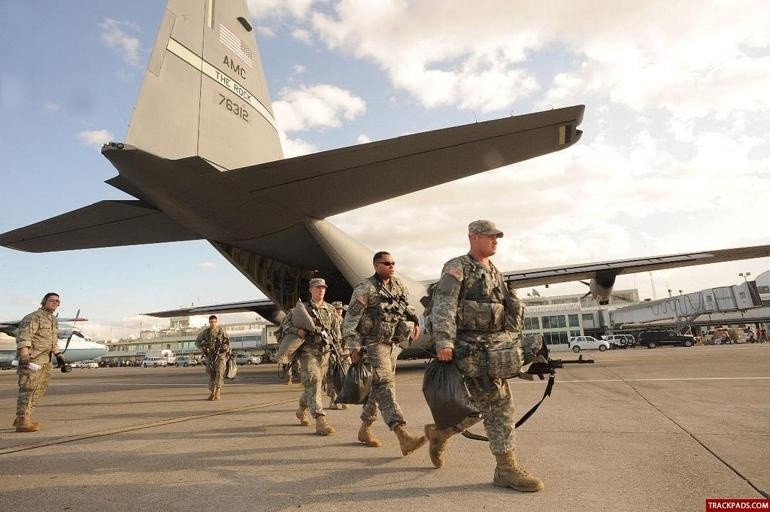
(161, 350), (177, 366)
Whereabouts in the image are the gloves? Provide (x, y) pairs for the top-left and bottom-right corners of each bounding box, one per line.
(55, 352), (65, 368)
(19, 354), (30, 370)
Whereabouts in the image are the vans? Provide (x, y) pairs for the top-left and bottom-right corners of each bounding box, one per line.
(743, 328), (758, 341)
(705, 330), (715, 343)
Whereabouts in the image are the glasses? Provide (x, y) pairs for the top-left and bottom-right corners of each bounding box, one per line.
(376, 262), (396, 266)
(49, 298), (60, 303)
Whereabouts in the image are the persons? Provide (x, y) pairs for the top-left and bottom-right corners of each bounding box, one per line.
(13, 293), (68, 432)
(98, 360), (142, 367)
(419, 220), (544, 492)
(343, 252), (428, 456)
(274, 304), (306, 385)
(282, 278), (346, 435)
(261, 353), (275, 364)
(330, 302), (348, 411)
(195, 315), (237, 400)
(191, 357), (201, 365)
(701, 321), (769, 345)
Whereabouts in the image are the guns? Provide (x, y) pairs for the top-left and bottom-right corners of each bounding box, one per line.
(462, 355), (595, 442)
(379, 281), (419, 324)
(309, 308), (346, 375)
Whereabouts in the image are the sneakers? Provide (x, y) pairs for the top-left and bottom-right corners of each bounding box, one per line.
(208, 391), (215, 400)
(296, 407), (312, 426)
(342, 403), (348, 410)
(286, 378), (293, 385)
(394, 425), (427, 456)
(13, 417), (40, 427)
(215, 389), (221, 400)
(358, 422), (382, 447)
(425, 424), (457, 468)
(316, 416), (335, 435)
(494, 448), (544, 492)
(330, 397), (338, 409)
(16, 415), (40, 432)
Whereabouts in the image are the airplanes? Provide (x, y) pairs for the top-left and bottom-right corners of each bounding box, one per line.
(0, 1), (770, 361)
(0, 309), (109, 373)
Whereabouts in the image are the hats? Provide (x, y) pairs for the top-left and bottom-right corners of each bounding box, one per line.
(332, 301), (344, 309)
(309, 279), (327, 288)
(469, 219), (504, 238)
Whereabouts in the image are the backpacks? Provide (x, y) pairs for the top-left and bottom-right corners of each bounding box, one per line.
(419, 281), (437, 354)
(225, 355), (237, 379)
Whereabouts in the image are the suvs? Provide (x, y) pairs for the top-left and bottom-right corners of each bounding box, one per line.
(625, 334), (636, 348)
(234, 354), (261, 365)
(174, 356), (197, 367)
(605, 334), (628, 349)
(141, 356), (167, 368)
(638, 330), (697, 349)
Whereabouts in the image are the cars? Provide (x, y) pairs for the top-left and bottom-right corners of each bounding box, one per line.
(77, 360), (98, 369)
(570, 336), (610, 353)
(69, 361), (80, 367)
